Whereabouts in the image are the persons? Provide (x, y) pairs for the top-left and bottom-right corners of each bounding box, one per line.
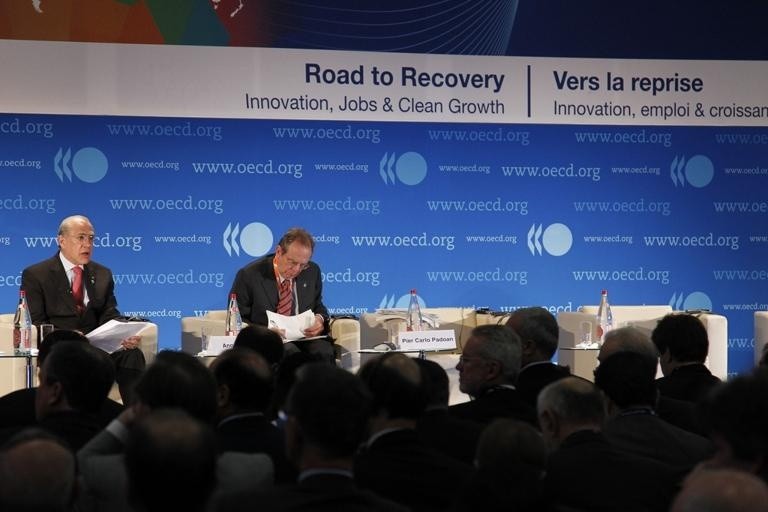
(225, 227), (336, 383)
(18, 215), (145, 408)
(0, 306), (765, 511)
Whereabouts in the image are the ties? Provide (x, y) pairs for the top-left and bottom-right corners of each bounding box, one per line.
(276, 279), (293, 316)
(70, 266), (86, 312)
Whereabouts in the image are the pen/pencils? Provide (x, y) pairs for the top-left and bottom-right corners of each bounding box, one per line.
(270, 320), (287, 340)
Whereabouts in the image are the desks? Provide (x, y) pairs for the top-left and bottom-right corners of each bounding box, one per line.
(355, 343), (458, 361)
(0, 347), (41, 388)
(557, 343), (603, 352)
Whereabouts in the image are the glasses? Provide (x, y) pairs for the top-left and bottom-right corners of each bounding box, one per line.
(286, 257), (310, 271)
(459, 355), (481, 368)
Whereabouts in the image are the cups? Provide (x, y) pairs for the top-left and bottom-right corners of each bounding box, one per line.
(40, 323), (54, 342)
(579, 320), (593, 346)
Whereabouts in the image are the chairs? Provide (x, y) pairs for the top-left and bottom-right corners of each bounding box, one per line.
(752, 311), (767, 370)
(360, 304), (510, 406)
(1, 312), (159, 406)
(183, 307), (360, 377)
(557, 303), (729, 389)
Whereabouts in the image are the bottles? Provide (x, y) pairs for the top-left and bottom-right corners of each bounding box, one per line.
(226, 293), (243, 336)
(597, 290), (614, 346)
(13, 290), (32, 355)
(406, 289), (424, 330)
